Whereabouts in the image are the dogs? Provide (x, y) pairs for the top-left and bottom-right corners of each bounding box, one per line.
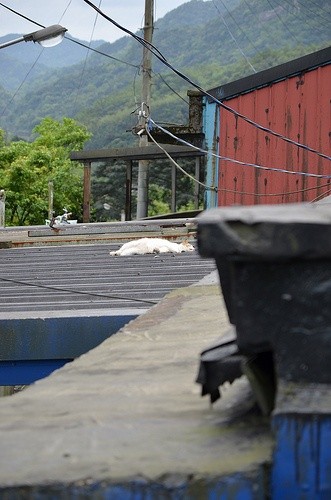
(110, 237), (195, 258)
